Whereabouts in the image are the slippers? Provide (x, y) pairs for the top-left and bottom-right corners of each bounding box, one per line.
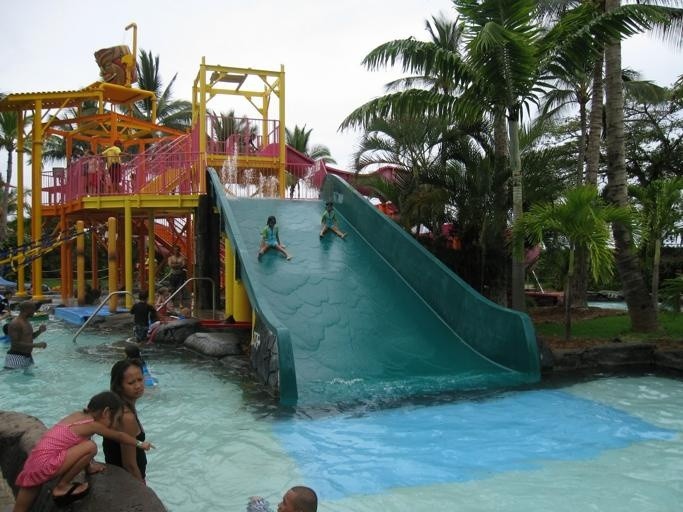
(51, 481), (91, 503)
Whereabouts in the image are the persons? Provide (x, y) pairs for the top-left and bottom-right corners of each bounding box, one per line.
(257, 216), (291, 261)
(154, 286), (178, 323)
(101, 358), (147, 486)
(167, 245), (185, 308)
(96, 139), (134, 195)
(318, 201), (347, 239)
(0, 298), (46, 368)
(125, 343), (157, 399)
(11, 390), (155, 512)
(82, 151), (100, 197)
(245, 485), (319, 512)
(129, 291), (158, 341)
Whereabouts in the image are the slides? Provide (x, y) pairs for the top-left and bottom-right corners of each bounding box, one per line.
(207, 162), (545, 410)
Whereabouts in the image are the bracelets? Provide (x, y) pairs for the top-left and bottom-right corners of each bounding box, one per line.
(135, 439), (141, 448)
